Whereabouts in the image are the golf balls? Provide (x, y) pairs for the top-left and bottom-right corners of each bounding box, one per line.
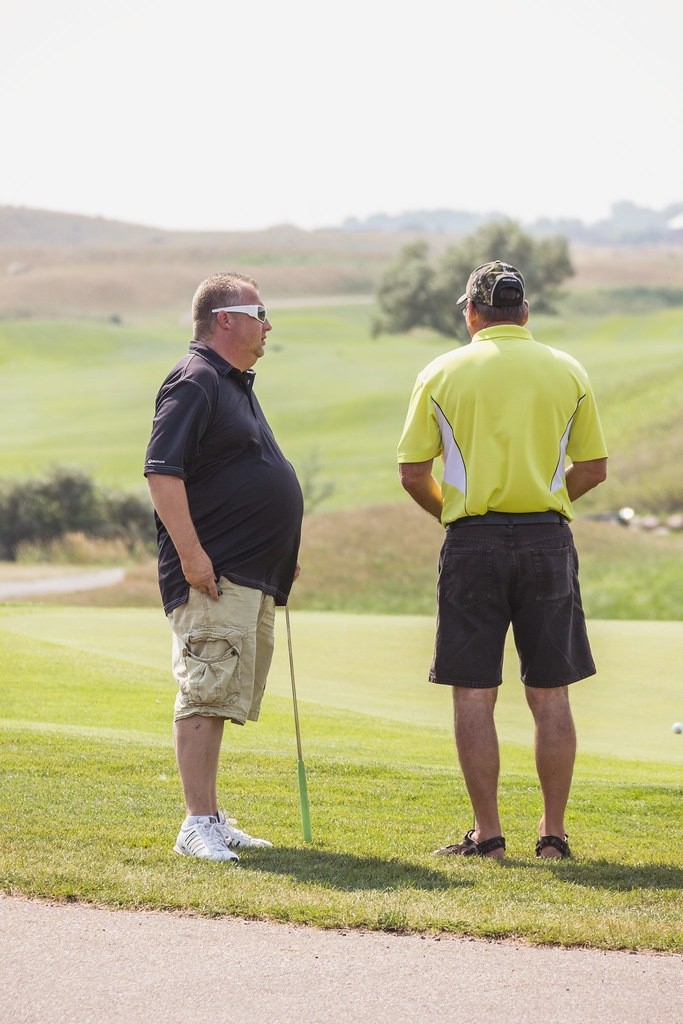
(672, 722), (683, 734)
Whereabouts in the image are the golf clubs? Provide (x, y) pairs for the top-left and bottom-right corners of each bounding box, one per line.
(285, 600), (313, 843)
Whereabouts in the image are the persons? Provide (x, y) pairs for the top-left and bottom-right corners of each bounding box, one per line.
(397, 260), (609, 867)
(143, 272), (305, 864)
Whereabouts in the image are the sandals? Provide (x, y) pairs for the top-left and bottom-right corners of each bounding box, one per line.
(433, 829), (506, 858)
(535, 834), (572, 861)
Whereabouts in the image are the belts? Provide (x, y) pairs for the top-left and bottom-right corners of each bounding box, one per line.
(449, 510), (569, 530)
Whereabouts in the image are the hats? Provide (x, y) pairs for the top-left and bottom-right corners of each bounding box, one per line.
(455, 260), (526, 306)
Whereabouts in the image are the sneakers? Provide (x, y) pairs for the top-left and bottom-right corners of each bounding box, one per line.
(172, 815), (240, 862)
(213, 810), (274, 849)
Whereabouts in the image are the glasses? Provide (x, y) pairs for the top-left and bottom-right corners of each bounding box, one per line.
(460, 302), (486, 317)
(210, 304), (267, 323)
